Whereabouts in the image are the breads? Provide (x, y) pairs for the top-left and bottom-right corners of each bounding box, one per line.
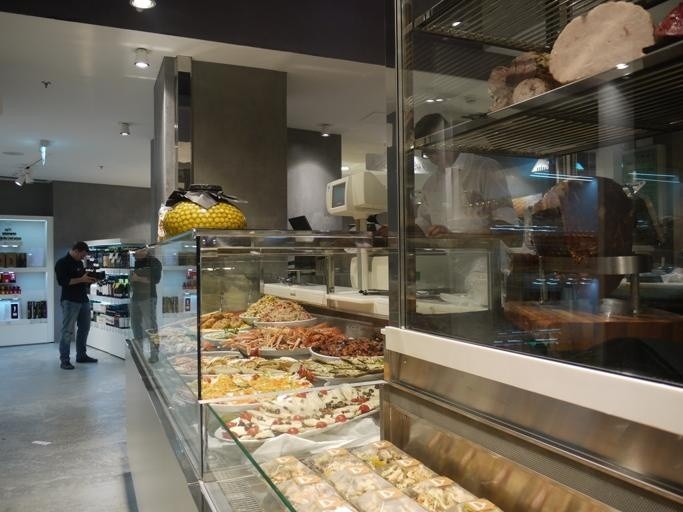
(199, 313), (244, 330)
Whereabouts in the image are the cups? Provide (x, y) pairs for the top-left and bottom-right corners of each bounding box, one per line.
(0, 227), (22, 241)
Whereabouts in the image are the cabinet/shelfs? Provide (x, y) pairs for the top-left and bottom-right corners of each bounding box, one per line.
(375, 0), (683, 512)
(75, 239), (146, 360)
(0, 214), (55, 347)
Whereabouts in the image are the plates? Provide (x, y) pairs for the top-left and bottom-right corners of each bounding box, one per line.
(151, 312), (382, 447)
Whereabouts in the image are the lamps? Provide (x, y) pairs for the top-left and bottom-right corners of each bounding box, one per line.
(130, 0), (158, 9)
(321, 124), (330, 137)
(135, 48), (149, 69)
(14, 158), (41, 187)
(120, 123), (130, 136)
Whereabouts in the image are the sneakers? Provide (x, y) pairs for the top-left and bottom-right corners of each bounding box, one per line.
(76, 353), (98, 362)
(60, 362), (75, 370)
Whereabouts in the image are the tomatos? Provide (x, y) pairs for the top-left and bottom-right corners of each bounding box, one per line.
(223, 387), (370, 442)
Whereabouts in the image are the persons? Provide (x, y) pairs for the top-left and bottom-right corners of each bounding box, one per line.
(379, 113), (517, 243)
(127, 244), (162, 364)
(54, 240), (98, 369)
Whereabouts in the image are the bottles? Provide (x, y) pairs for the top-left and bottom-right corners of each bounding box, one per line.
(89, 248), (131, 328)
(0, 284), (22, 294)
